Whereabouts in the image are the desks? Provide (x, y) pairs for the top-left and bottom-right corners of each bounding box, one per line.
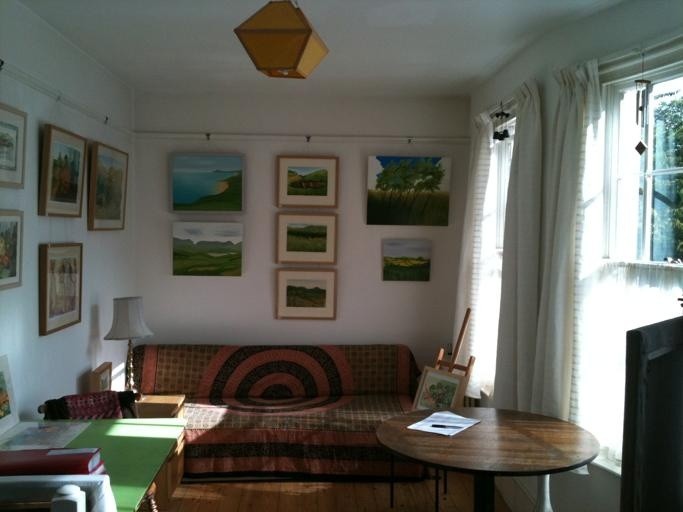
(1, 418), (190, 512)
(374, 407), (600, 509)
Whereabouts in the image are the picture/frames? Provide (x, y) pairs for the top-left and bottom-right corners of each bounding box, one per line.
(39, 243), (81, 334)
(0, 355), (17, 436)
(0, 208), (22, 290)
(276, 212), (338, 264)
(167, 150), (247, 218)
(38, 123), (89, 218)
(277, 269), (336, 320)
(275, 154), (338, 209)
(0, 100), (26, 190)
(86, 138), (129, 230)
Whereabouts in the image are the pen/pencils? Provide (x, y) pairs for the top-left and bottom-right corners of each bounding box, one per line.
(432, 425), (462, 428)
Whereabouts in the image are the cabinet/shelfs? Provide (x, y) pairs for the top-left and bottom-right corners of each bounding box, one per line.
(129, 394), (185, 494)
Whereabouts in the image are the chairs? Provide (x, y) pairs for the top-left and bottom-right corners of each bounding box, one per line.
(36, 390), (161, 512)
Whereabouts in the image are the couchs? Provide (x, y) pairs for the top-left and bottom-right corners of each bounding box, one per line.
(129, 342), (436, 484)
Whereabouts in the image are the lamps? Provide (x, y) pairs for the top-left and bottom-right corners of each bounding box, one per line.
(231, 0), (330, 80)
(103, 296), (153, 401)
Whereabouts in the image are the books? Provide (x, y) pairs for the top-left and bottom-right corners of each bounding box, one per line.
(88, 464), (107, 478)
(0, 446), (101, 474)
